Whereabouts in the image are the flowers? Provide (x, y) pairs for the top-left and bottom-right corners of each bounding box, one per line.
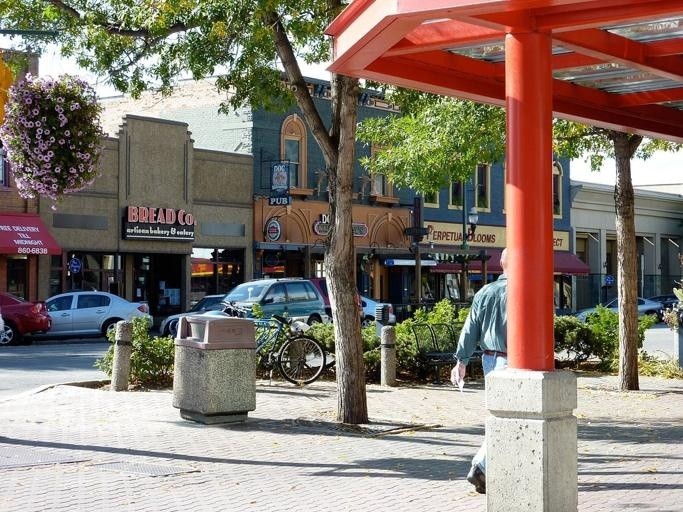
(0, 71), (109, 210)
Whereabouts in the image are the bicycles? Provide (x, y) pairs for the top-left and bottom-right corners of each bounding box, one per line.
(222, 300), (325, 384)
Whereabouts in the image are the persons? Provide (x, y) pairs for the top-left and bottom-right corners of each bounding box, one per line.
(448, 248), (507, 494)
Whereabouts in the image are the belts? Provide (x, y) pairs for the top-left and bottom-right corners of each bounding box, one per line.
(482, 349), (507, 357)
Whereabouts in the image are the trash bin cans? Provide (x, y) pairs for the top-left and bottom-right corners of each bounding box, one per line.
(173, 315), (257, 425)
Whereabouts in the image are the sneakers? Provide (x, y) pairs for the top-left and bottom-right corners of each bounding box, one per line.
(465, 466), (486, 495)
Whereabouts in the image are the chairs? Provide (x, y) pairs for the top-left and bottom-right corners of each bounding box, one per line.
(411, 322), (482, 383)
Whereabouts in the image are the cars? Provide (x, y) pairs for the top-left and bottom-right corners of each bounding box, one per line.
(359, 295), (396, 325)
(22, 291), (153, 341)
(576, 295), (680, 323)
(1, 291), (51, 345)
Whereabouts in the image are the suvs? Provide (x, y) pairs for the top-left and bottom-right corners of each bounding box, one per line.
(159, 276), (332, 335)
